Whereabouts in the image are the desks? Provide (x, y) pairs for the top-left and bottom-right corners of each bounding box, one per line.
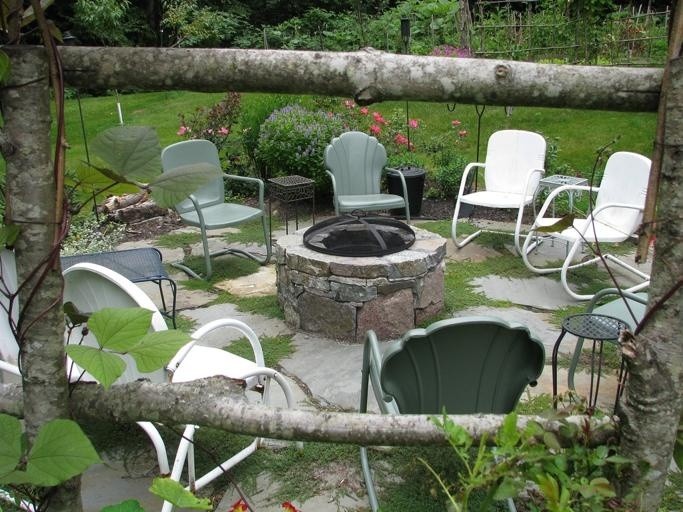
(550, 313), (633, 417)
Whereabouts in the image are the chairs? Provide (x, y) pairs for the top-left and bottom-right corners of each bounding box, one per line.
(64, 262), (307, 512)
(0, 246), (171, 480)
(358, 315), (546, 512)
(159, 138), (270, 282)
(568, 287), (646, 409)
(323, 132), (412, 223)
(56, 247), (178, 329)
(450, 130), (547, 260)
(521, 150), (652, 301)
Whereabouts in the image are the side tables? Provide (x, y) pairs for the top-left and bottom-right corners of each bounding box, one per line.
(534, 173), (590, 248)
(269, 175), (316, 233)
